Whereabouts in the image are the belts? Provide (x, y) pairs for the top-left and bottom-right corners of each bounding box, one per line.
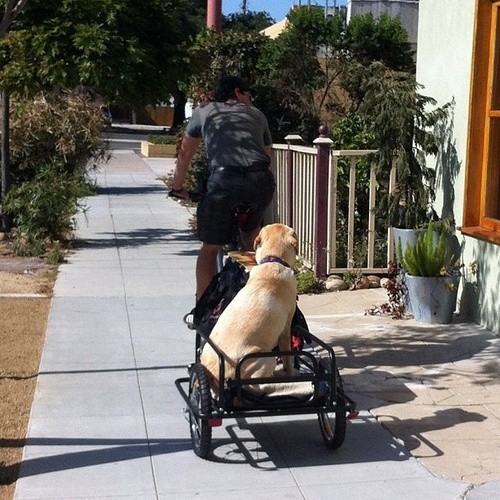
(213, 162), (269, 173)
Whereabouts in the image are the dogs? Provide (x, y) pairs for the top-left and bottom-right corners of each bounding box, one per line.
(200, 223), (299, 403)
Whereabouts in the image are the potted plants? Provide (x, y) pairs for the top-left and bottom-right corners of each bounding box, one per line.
(355, 60), (453, 281)
(395, 219), (465, 324)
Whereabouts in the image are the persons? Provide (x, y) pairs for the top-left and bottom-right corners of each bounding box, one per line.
(170, 75), (278, 307)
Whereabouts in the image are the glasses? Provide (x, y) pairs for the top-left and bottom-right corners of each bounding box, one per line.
(242, 91), (253, 103)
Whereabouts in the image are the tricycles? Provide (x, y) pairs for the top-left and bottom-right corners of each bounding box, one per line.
(167, 190), (354, 457)
